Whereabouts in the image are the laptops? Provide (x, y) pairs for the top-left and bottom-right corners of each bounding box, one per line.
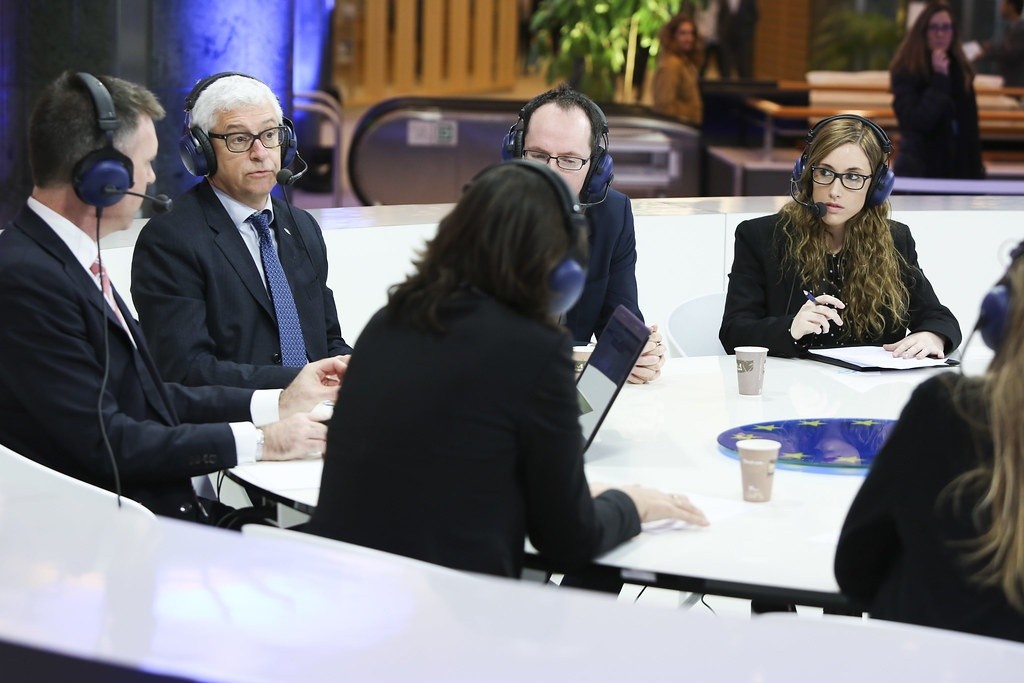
(577, 305), (650, 455)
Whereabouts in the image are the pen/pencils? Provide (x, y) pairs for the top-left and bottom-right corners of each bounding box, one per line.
(803, 290), (821, 305)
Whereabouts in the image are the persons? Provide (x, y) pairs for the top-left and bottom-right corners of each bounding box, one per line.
(502, 82), (668, 385)
(0, 72), (348, 531)
(834, 232), (1024, 644)
(650, 0), (760, 123)
(888, 0), (1024, 179)
(130, 73), (358, 390)
(308, 159), (710, 582)
(718, 114), (962, 360)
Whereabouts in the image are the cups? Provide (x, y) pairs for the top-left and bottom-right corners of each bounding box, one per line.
(737, 439), (782, 504)
(734, 347), (770, 395)
(572, 345), (597, 381)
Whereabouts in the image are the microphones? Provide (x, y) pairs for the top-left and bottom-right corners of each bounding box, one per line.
(791, 181), (827, 218)
(276, 155), (308, 185)
(574, 186), (609, 213)
(116, 190), (174, 215)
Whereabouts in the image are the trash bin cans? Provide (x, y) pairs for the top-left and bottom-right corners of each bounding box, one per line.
(699, 80), (778, 197)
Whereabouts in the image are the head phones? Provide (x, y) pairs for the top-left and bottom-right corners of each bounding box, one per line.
(502, 100), (616, 205)
(792, 113), (895, 208)
(70, 72), (135, 216)
(460, 159), (586, 317)
(978, 239), (1024, 353)
(178, 72), (298, 177)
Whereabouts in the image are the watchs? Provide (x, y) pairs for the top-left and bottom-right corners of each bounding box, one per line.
(256, 428), (265, 462)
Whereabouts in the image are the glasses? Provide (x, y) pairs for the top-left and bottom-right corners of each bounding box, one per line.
(927, 23), (952, 32)
(208, 124), (287, 153)
(522, 150), (592, 170)
(811, 165), (872, 190)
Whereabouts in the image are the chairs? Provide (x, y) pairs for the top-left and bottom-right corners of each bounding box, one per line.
(661, 290), (726, 358)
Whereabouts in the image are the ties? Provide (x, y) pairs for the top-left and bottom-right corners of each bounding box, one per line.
(90, 257), (138, 352)
(245, 211), (308, 368)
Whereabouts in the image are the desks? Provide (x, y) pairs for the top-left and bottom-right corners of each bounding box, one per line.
(221, 353), (989, 611)
